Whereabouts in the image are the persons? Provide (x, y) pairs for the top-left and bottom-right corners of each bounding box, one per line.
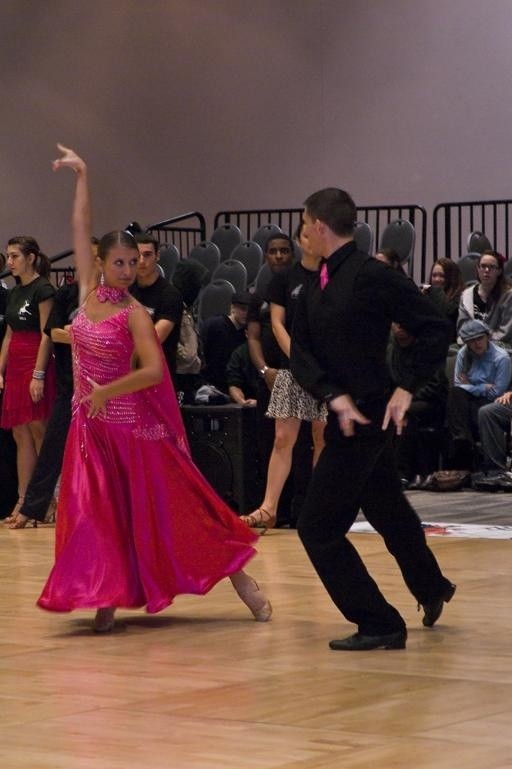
(33, 138), (272, 638)
(2, 221), (326, 537)
(374, 250), (511, 491)
(288, 186), (458, 654)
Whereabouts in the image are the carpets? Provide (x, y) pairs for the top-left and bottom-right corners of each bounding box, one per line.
(346, 475), (512, 540)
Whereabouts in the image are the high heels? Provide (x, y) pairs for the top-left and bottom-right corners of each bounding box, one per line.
(6, 499), (57, 529)
(239, 509), (277, 535)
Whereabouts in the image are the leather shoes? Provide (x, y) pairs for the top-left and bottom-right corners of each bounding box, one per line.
(329, 627), (407, 649)
(419, 580), (456, 626)
(400, 467), (511, 491)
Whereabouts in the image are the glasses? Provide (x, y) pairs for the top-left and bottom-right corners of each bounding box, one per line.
(480, 263), (500, 271)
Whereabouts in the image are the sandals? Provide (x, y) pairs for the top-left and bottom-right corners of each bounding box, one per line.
(96, 608), (115, 631)
(239, 577), (272, 622)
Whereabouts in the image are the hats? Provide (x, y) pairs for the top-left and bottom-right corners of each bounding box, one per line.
(458, 320), (491, 342)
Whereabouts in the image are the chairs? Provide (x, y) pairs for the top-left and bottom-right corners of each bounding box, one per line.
(251, 224), (283, 252)
(159, 242), (180, 283)
(352, 221), (374, 258)
(426, 228), (511, 385)
(254, 262), (273, 297)
(211, 257), (247, 295)
(380, 218), (417, 265)
(172, 261), (210, 307)
(199, 279), (236, 326)
(210, 223), (243, 262)
(228, 241), (264, 286)
(189, 242), (223, 275)
